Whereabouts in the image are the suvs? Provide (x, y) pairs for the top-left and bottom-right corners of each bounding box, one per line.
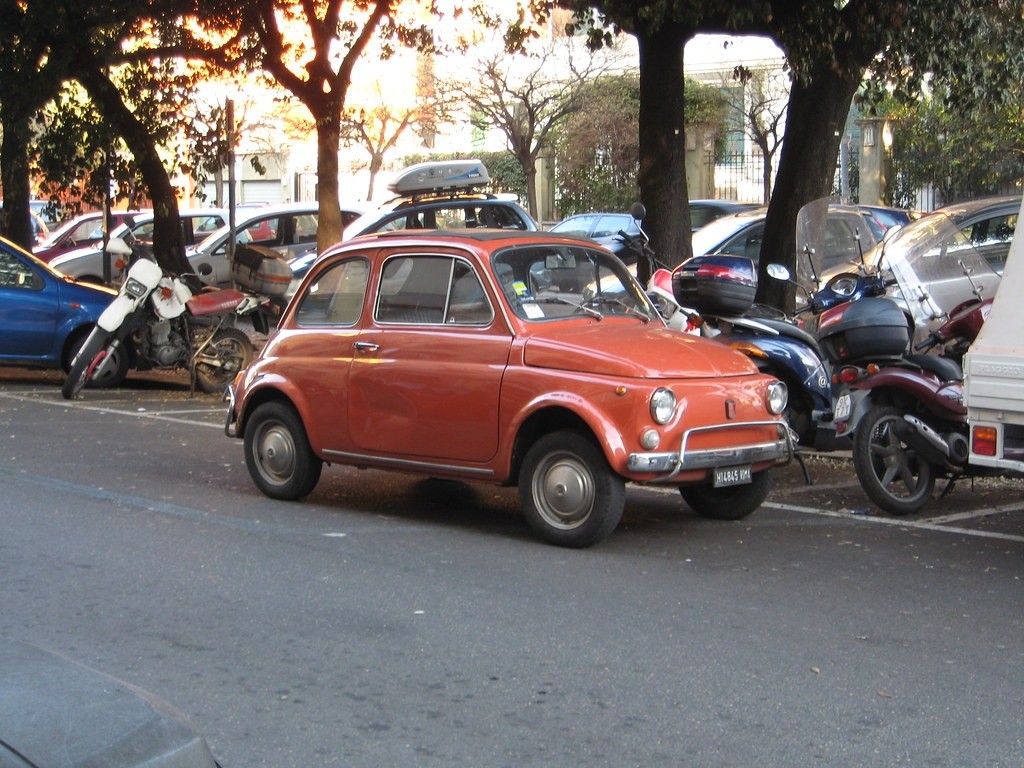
(269, 160), (547, 312)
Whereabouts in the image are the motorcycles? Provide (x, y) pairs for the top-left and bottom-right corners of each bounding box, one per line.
(63, 220), (291, 400)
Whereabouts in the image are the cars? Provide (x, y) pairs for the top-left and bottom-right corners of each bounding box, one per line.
(51, 208), (233, 286)
(579, 203), (876, 322)
(545, 209), (643, 294)
(183, 203), (401, 296)
(684, 197), (763, 230)
(0, 238), (142, 389)
(811, 200), (1024, 351)
(29, 208), (157, 269)
(236, 225), (798, 547)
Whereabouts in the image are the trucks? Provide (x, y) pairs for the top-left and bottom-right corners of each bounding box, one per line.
(960, 197), (1023, 474)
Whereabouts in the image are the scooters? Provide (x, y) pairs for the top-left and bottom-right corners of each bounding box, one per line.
(620, 193), (1006, 515)
